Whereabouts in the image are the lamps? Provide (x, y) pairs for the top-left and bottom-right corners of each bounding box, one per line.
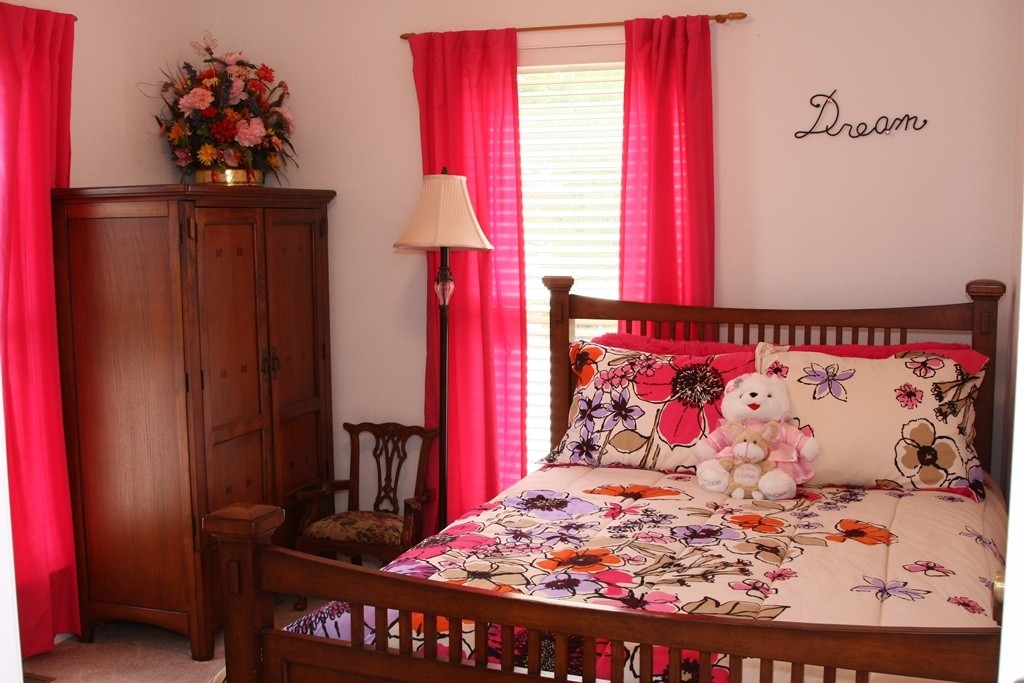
(392, 161), (495, 533)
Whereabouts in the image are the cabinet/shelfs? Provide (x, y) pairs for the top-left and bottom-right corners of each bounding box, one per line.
(48, 186), (335, 661)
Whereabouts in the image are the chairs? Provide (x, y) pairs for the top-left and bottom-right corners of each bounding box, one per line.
(293, 421), (440, 566)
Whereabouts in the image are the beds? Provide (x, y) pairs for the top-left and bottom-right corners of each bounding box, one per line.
(201, 275), (1007, 682)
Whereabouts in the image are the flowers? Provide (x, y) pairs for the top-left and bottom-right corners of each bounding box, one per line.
(135, 30), (299, 189)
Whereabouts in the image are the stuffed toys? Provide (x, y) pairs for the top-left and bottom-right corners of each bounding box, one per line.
(694, 372), (820, 501)
(717, 419), (780, 501)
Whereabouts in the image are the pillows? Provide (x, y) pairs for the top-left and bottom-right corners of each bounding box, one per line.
(532, 341), (758, 468)
(749, 343), (989, 501)
